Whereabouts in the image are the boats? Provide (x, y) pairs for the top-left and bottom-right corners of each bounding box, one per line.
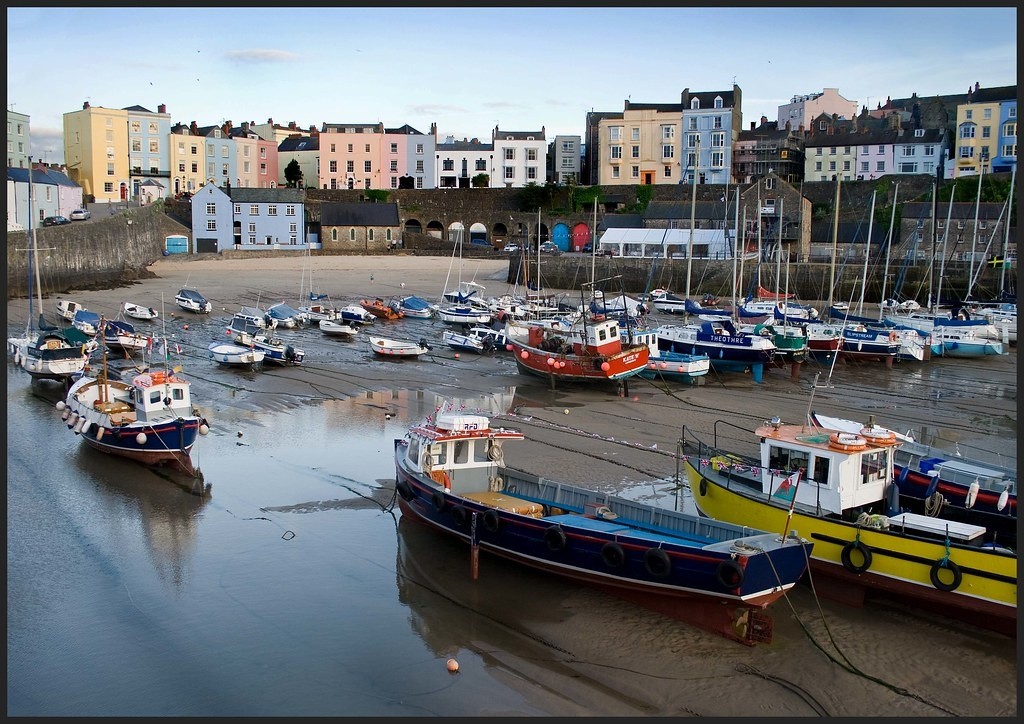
(674, 413), (1019, 640)
(55, 299), (88, 321)
(103, 303), (147, 351)
(392, 410), (813, 610)
(124, 302), (159, 320)
(57, 312), (210, 468)
(805, 409), (1020, 549)
(175, 287), (213, 314)
(72, 308), (103, 335)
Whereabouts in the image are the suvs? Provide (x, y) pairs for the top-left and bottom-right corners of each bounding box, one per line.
(42, 215), (71, 227)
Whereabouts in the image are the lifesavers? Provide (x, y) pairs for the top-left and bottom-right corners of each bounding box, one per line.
(427, 470), (452, 492)
(150, 372), (166, 383)
(591, 314), (605, 321)
(889, 332), (897, 341)
(828, 429), (896, 451)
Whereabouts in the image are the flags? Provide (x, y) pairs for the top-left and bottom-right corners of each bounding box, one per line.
(73, 315), (182, 379)
(772, 470), (800, 502)
(399, 399), (781, 480)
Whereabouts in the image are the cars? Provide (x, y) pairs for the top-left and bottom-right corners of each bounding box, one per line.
(71, 209), (91, 220)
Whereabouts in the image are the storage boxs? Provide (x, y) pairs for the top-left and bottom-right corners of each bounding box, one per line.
(439, 415), (490, 432)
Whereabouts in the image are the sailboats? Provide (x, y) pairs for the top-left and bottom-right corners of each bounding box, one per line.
(212, 173), (1019, 391)
(8, 156), (98, 376)
